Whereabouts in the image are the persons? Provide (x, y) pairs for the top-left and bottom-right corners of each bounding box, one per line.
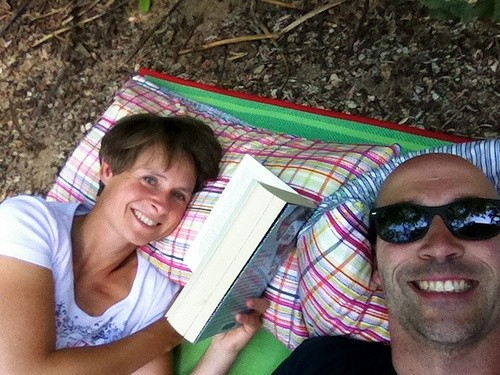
(2, 112), (275, 375)
(272, 154), (500, 374)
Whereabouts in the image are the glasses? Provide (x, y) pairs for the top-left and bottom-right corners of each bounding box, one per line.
(367, 199), (500, 244)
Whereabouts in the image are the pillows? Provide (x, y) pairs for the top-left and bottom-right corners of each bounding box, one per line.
(46, 73), (401, 352)
(297, 139), (500, 346)
